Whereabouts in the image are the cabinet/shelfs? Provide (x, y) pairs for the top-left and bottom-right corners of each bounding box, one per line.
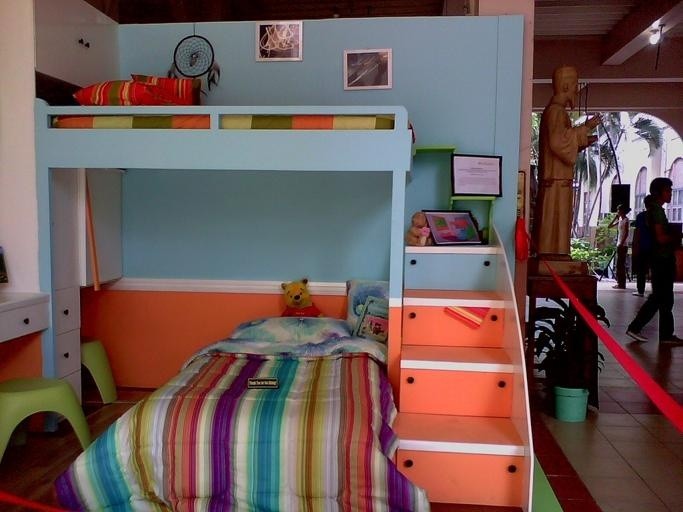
(55, 286), (82, 406)
(0, 302), (50, 343)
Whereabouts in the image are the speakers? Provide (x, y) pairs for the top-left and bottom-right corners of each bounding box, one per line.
(610, 184), (630, 214)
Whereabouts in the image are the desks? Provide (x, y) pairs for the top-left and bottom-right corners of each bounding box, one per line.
(526, 260), (600, 392)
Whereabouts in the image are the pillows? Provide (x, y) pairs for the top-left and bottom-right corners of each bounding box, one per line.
(74, 80), (171, 105)
(131, 74), (201, 105)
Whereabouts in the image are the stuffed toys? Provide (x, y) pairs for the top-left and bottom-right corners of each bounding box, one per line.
(405, 211), (434, 247)
(281, 278), (324, 319)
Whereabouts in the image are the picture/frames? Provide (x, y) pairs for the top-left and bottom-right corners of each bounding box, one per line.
(343, 48), (393, 91)
(451, 154), (502, 197)
(421, 210), (485, 245)
(254, 21), (303, 62)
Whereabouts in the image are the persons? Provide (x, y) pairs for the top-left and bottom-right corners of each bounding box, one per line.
(625, 177), (683, 345)
(532, 64), (605, 261)
(631, 195), (653, 297)
(607, 203), (630, 289)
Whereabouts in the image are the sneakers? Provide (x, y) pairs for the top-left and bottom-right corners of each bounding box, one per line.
(662, 335), (683, 344)
(611, 285), (625, 289)
(626, 329), (648, 342)
(632, 291), (644, 296)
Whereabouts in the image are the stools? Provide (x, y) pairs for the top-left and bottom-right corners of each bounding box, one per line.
(0, 378), (92, 465)
(81, 336), (118, 404)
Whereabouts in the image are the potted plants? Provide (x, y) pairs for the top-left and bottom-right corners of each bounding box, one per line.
(526, 296), (610, 418)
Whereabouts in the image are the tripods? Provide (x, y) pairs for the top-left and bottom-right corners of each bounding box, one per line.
(598, 214), (631, 282)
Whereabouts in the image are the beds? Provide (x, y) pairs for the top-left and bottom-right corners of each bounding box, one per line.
(36, 98), (534, 511)
(55, 317), (432, 512)
